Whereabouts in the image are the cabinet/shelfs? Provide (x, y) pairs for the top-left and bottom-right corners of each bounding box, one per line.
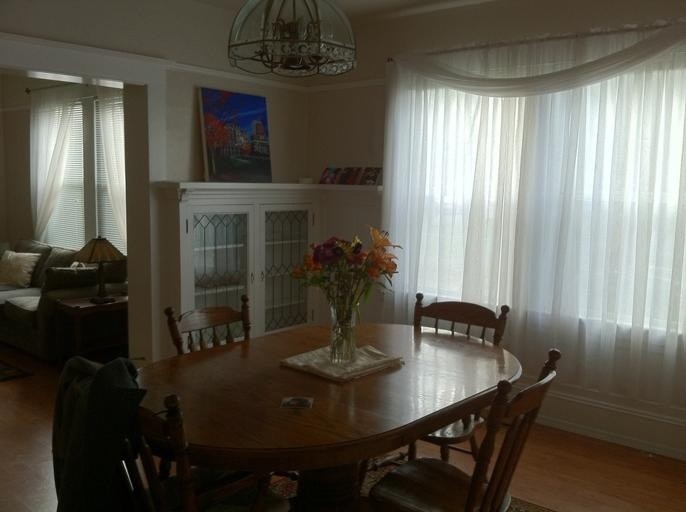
(152, 180), (384, 353)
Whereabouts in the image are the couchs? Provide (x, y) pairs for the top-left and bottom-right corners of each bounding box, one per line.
(0, 236), (128, 360)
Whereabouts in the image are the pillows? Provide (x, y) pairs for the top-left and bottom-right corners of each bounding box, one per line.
(0, 249), (42, 288)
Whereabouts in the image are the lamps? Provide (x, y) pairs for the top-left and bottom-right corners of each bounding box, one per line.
(227, 0), (358, 78)
(71, 235), (128, 304)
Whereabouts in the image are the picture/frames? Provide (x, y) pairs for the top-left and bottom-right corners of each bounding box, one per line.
(197, 86), (273, 184)
(313, 165), (383, 186)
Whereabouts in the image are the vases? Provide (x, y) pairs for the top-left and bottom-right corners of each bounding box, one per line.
(327, 294), (360, 366)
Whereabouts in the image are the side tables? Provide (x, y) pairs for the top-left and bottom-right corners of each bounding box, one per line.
(47, 292), (128, 355)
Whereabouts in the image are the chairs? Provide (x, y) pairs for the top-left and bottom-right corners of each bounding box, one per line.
(407, 293), (510, 484)
(50, 356), (262, 512)
(163, 294), (253, 356)
(367, 346), (563, 512)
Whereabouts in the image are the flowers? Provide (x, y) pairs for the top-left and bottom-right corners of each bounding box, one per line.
(290, 223), (404, 305)
